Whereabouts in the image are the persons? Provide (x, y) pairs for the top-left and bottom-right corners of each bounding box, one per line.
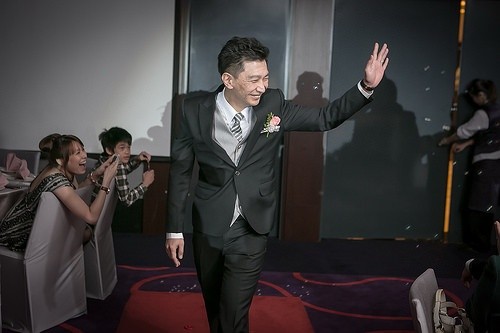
(0, 126), (155, 254)
(437, 78), (500, 333)
(165, 36), (390, 333)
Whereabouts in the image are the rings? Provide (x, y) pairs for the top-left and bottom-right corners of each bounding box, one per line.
(380, 61), (383, 63)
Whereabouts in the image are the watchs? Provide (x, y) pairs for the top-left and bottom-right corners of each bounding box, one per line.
(101, 186), (111, 194)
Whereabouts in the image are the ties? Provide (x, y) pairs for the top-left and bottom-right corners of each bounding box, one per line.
(231, 113), (244, 143)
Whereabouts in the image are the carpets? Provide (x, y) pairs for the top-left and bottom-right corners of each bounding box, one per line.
(45, 266), (481, 333)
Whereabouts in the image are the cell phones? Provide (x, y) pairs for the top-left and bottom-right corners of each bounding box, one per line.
(147, 160), (150, 172)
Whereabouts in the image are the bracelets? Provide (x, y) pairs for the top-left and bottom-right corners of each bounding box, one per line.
(87, 172), (96, 183)
(360, 81), (377, 91)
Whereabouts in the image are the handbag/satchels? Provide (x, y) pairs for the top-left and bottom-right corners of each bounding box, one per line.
(433, 289), (475, 333)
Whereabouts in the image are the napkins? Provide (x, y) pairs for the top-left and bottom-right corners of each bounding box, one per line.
(0, 172), (9, 191)
(5, 151), (21, 171)
(15, 159), (30, 181)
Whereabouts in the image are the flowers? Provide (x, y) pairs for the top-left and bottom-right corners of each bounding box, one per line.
(261, 111), (281, 137)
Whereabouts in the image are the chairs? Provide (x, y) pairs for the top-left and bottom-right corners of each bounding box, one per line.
(410, 267), (448, 333)
(0, 150), (144, 333)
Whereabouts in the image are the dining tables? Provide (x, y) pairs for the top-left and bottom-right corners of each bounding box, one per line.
(0, 166), (37, 223)
